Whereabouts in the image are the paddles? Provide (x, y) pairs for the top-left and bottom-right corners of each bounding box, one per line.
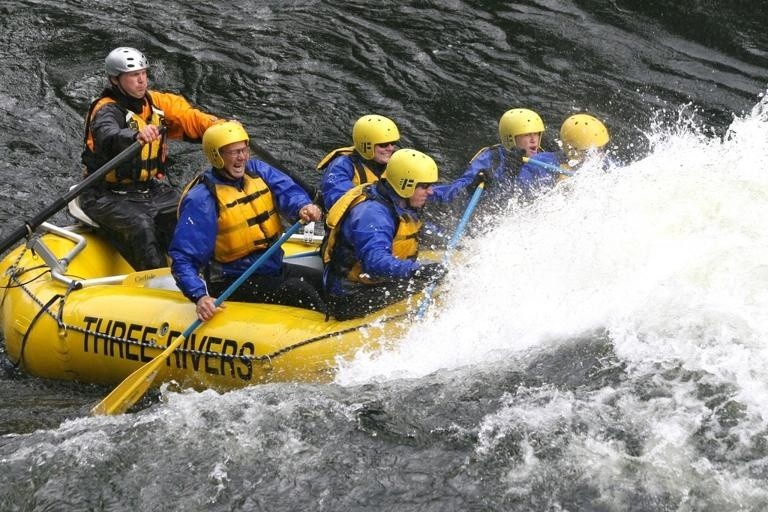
(91, 210), (310, 413)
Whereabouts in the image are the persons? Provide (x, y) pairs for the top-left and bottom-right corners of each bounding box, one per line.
(518, 113), (615, 196)
(167, 120), (323, 321)
(316, 115), (400, 258)
(430, 108), (546, 203)
(78, 46), (243, 271)
(322, 147), (449, 322)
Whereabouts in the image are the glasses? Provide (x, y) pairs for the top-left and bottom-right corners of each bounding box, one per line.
(379, 142), (396, 147)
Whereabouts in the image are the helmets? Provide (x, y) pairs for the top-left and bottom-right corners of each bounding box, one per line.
(104, 47), (149, 76)
(560, 114), (609, 164)
(381, 149), (438, 198)
(499, 109), (545, 152)
(352, 115), (400, 160)
(202, 122), (249, 169)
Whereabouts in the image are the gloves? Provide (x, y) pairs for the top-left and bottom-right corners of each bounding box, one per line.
(468, 167), (493, 194)
(415, 264), (448, 282)
(500, 146), (527, 176)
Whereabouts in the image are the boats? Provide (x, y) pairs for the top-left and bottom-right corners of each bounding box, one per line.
(0, 220), (464, 391)
